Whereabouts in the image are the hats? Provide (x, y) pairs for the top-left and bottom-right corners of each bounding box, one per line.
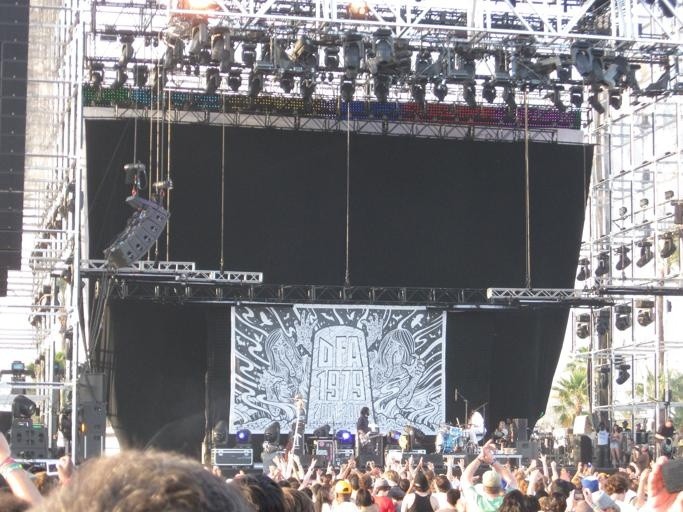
(483, 470), (501, 488)
(335, 480), (352, 494)
(414, 473), (428, 487)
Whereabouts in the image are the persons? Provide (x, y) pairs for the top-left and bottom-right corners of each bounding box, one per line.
(356, 407), (373, 473)
(467, 408), (485, 447)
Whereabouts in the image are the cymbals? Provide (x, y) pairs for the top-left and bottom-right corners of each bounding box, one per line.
(463, 423), (474, 424)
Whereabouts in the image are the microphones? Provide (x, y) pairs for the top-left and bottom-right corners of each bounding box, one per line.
(452, 387), (458, 401)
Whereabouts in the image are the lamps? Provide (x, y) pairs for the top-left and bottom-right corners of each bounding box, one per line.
(211, 419), (229, 449)
(14, 395), (36, 416)
(28, 178), (83, 328)
(89, 1), (682, 115)
(124, 162), (172, 207)
(314, 424), (330, 438)
(265, 422), (281, 447)
(575, 230), (676, 389)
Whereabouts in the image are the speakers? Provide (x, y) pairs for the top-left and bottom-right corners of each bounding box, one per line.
(71, 371), (108, 467)
(356, 435), (384, 467)
(512, 418), (528, 441)
(102, 206), (171, 268)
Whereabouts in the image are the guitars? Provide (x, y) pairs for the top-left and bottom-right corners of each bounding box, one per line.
(359, 431), (382, 449)
